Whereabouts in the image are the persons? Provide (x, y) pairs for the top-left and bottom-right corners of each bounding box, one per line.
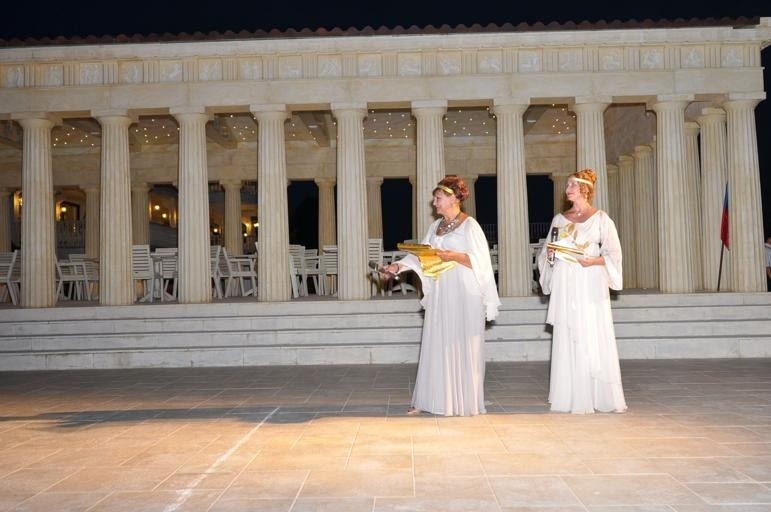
(536, 169), (624, 413)
(381, 175), (493, 417)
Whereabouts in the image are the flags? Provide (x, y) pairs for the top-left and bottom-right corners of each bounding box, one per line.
(720, 182), (732, 250)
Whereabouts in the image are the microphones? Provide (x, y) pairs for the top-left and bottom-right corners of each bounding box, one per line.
(549, 227), (558, 267)
(368, 261), (400, 282)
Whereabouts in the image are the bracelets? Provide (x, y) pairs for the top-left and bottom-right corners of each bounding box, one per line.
(396, 264), (400, 273)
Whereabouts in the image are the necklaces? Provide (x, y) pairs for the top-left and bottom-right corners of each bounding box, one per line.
(440, 211), (462, 235)
(573, 205), (590, 216)
